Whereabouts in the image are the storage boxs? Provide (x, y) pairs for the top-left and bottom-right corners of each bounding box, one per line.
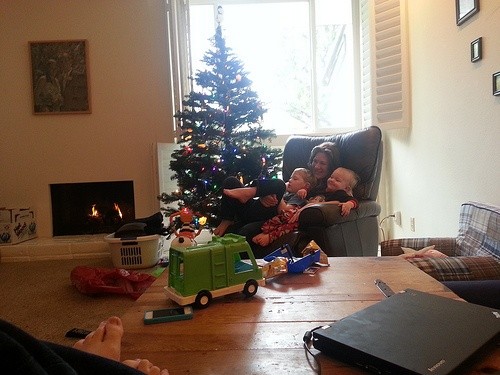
(104, 232), (164, 269)
(0, 206), (38, 245)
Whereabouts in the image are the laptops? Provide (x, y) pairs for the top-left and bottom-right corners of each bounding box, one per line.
(311, 287), (500, 375)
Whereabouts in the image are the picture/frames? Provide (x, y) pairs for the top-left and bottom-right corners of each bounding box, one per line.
(471, 37), (482, 62)
(492, 70), (500, 96)
(27, 38), (92, 115)
(455, 0), (479, 26)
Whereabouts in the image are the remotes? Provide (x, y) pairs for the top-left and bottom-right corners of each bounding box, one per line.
(65, 327), (92, 338)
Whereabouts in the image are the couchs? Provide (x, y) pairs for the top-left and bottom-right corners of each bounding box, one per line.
(229, 124), (384, 257)
(381, 201), (500, 281)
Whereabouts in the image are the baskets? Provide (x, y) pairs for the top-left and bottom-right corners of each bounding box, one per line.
(104, 232), (164, 269)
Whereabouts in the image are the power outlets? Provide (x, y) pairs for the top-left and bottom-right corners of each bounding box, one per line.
(392, 211), (401, 226)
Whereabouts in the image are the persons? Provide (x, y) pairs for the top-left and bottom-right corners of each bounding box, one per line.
(35, 60), (65, 112)
(215, 142), (360, 247)
(0, 315), (169, 375)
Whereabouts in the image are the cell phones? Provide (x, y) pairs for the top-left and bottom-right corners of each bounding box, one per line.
(143, 305), (193, 324)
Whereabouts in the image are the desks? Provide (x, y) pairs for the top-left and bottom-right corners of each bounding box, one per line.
(119, 257), (500, 374)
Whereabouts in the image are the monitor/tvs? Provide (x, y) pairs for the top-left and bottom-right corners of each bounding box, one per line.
(47, 179), (136, 242)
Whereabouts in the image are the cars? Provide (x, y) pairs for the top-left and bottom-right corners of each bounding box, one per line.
(164, 233), (265, 310)
